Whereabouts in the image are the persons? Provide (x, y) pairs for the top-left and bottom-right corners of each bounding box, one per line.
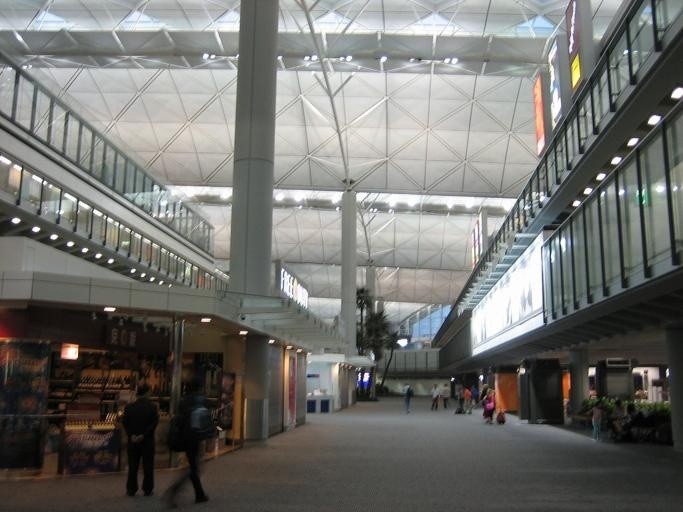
(401, 383), (411, 413)
(429, 381), (496, 425)
(164, 381), (212, 509)
(584, 386), (648, 447)
(219, 372), (235, 429)
(122, 383), (155, 497)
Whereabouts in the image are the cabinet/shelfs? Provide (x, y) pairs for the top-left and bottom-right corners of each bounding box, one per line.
(46, 350), (85, 428)
(72, 346), (139, 419)
(0, 336), (49, 474)
(55, 418), (121, 478)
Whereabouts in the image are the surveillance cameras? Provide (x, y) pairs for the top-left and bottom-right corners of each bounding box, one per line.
(240, 315), (246, 320)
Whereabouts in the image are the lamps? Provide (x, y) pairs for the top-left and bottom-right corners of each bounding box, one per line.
(58, 342), (80, 362)
